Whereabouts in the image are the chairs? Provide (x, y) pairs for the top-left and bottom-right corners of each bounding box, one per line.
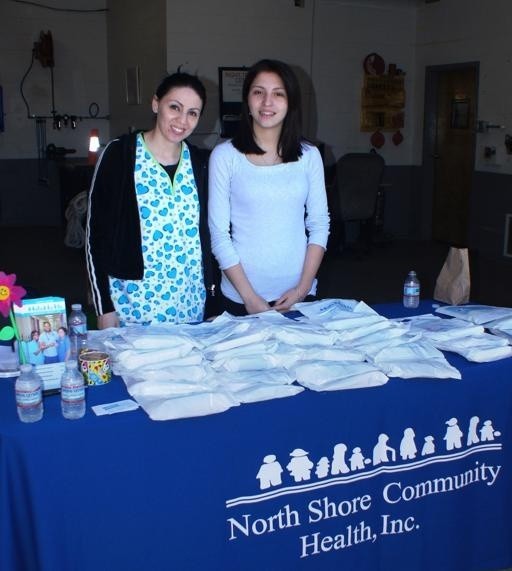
(326, 154), (385, 257)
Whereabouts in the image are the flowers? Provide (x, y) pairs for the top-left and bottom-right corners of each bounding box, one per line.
(0, 270), (28, 368)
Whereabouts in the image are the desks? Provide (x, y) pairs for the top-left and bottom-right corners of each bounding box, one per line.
(1, 300), (511, 525)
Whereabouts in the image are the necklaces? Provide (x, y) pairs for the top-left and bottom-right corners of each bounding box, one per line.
(263, 153), (280, 167)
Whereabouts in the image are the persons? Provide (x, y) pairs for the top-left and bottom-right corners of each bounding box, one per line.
(38, 322), (60, 365)
(83, 72), (220, 330)
(28, 330), (47, 367)
(55, 326), (73, 362)
(202, 59), (332, 316)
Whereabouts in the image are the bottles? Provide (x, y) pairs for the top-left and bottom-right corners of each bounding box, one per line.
(60, 360), (86, 421)
(15, 364), (45, 424)
(403, 271), (420, 309)
(67, 304), (88, 360)
